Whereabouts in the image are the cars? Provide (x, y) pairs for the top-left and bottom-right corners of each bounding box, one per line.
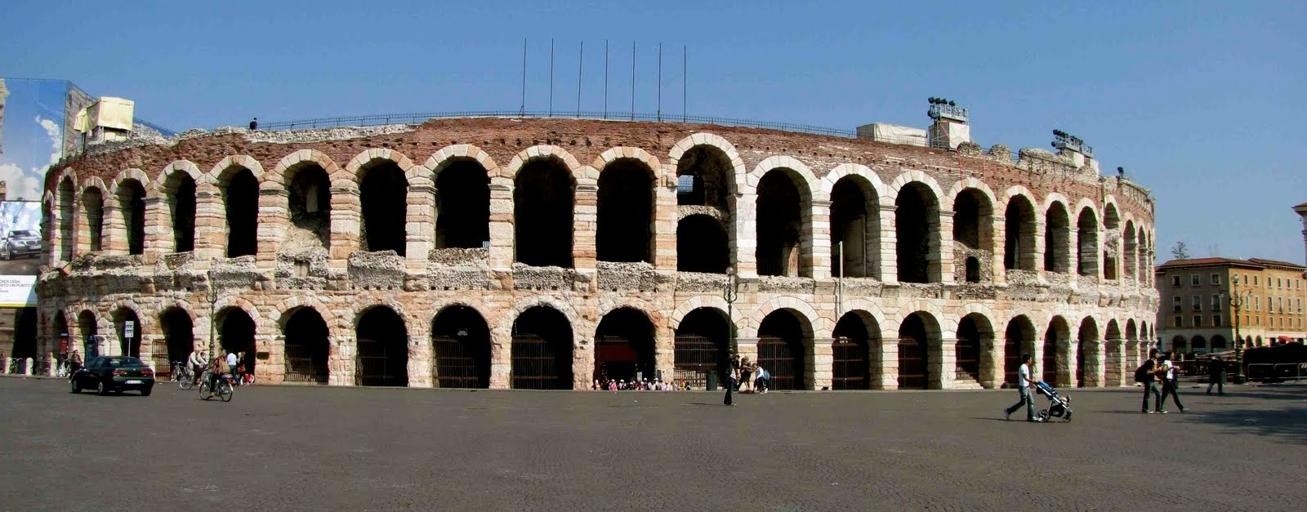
(0, 230), (43, 260)
(69, 355), (157, 398)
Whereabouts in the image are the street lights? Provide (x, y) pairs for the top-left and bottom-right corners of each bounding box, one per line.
(1228, 272), (1249, 385)
(723, 264), (739, 365)
(206, 268), (218, 368)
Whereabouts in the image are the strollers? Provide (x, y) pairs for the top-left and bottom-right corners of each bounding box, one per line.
(1033, 382), (1075, 423)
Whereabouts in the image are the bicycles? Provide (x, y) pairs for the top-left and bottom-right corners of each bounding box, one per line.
(196, 368), (234, 402)
(179, 364), (208, 391)
(169, 359), (185, 382)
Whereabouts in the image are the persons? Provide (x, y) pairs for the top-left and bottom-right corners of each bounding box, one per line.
(723, 348), (771, 407)
(1003, 354), (1040, 422)
(1159, 351), (1189, 412)
(186, 342), (251, 399)
(0, 349), (82, 384)
(1140, 348), (1168, 414)
(250, 117), (257, 130)
(1206, 355), (1226, 396)
(594, 374), (692, 392)
